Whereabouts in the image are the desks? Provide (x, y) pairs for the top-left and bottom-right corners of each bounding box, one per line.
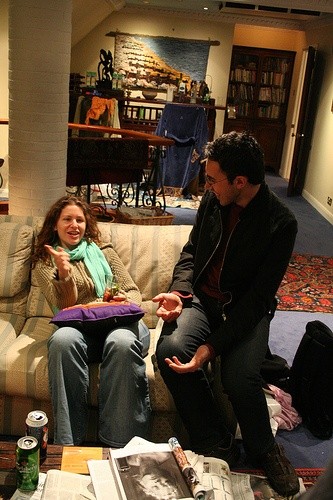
(0, 441), (120, 500)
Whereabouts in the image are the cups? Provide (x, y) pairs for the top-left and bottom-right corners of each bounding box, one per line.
(103, 274), (123, 302)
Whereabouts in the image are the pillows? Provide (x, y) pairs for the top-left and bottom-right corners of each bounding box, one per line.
(48, 301), (148, 334)
(26, 216), (109, 317)
(0, 221), (33, 298)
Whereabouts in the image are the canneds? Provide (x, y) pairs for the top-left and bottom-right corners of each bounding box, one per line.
(24, 410), (49, 461)
(15, 436), (40, 492)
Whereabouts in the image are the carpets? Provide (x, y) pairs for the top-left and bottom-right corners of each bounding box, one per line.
(274, 253), (333, 313)
(230, 468), (326, 500)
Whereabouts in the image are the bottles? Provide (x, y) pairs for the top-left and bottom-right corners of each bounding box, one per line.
(203, 84), (210, 103)
(189, 84), (197, 103)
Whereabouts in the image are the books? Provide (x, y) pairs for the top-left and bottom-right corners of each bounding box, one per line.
(10, 436), (307, 500)
(227, 55), (289, 118)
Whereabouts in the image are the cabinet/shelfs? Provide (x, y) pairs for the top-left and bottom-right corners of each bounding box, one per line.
(222, 45), (298, 177)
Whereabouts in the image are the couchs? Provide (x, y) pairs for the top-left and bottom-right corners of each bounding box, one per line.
(0, 215), (239, 452)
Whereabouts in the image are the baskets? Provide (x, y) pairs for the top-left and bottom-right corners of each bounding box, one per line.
(115, 205), (174, 225)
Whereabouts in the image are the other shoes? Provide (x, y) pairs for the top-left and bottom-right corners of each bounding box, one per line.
(262, 451), (300, 496)
(198, 433), (236, 460)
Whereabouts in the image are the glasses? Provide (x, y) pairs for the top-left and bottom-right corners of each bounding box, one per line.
(205, 176), (236, 185)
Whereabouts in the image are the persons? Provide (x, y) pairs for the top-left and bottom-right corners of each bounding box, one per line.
(126, 451), (183, 499)
(152, 131), (300, 498)
(32, 195), (150, 446)
